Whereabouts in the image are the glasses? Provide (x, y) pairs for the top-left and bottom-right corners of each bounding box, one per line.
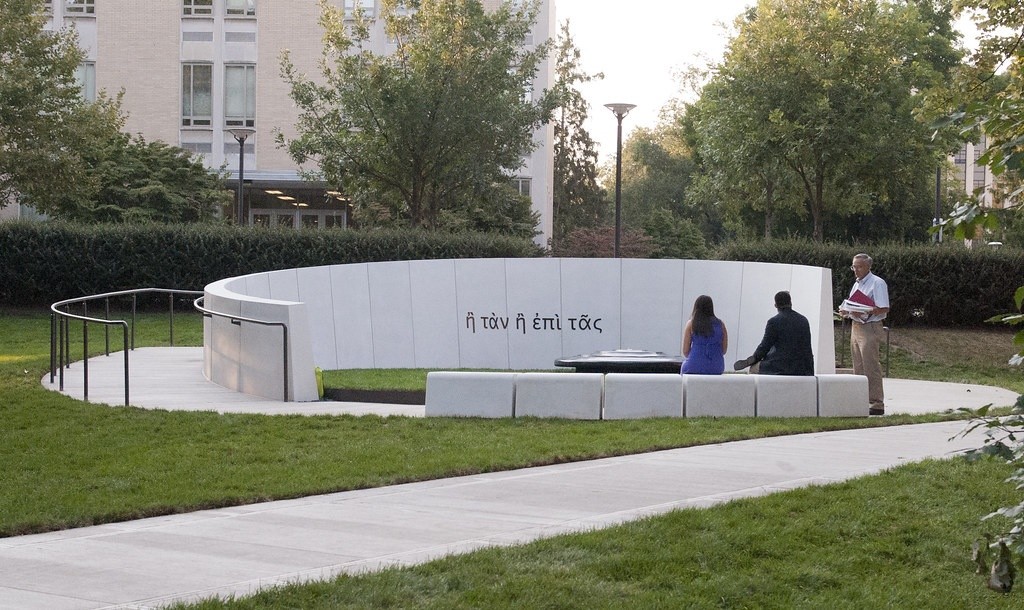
(850, 265), (865, 271)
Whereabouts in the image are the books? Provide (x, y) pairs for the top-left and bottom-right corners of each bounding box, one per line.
(839, 289), (875, 325)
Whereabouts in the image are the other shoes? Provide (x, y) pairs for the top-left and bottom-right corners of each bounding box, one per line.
(869, 408), (884, 415)
(734, 356), (755, 370)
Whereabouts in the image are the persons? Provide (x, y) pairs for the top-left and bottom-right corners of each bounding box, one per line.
(839, 253), (890, 414)
(680, 295), (728, 375)
(734, 291), (815, 376)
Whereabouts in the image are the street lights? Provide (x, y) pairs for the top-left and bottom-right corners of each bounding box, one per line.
(604, 103), (636, 260)
(226, 128), (256, 227)
